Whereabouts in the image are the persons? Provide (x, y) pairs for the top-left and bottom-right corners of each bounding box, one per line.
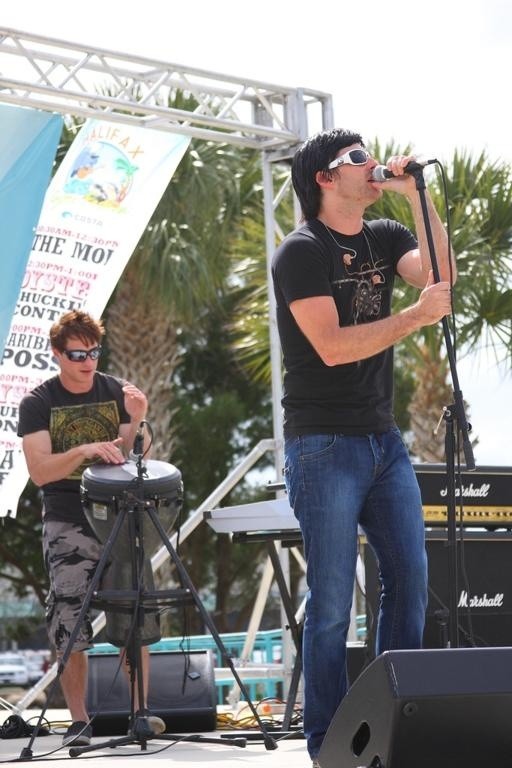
(16, 311), (167, 744)
(271, 131), (458, 767)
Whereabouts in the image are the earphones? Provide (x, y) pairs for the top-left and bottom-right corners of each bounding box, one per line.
(343, 253), (354, 266)
(372, 275), (382, 286)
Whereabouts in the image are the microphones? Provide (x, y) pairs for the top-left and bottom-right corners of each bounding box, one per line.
(372, 160), (429, 183)
(129, 427), (145, 462)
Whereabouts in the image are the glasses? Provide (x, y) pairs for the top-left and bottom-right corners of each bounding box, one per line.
(58, 345), (104, 363)
(324, 147), (372, 170)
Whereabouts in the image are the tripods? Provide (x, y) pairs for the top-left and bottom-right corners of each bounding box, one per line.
(20, 497), (279, 760)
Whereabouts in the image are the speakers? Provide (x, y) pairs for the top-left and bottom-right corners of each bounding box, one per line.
(313, 646), (512, 768)
(79, 649), (220, 731)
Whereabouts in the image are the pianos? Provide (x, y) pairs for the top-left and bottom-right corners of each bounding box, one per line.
(201, 497), (368, 534)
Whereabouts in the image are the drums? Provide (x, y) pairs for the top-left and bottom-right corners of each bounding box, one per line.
(81, 456), (183, 647)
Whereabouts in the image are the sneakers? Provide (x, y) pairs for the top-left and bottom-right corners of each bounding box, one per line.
(125, 708), (166, 738)
(60, 720), (95, 747)
